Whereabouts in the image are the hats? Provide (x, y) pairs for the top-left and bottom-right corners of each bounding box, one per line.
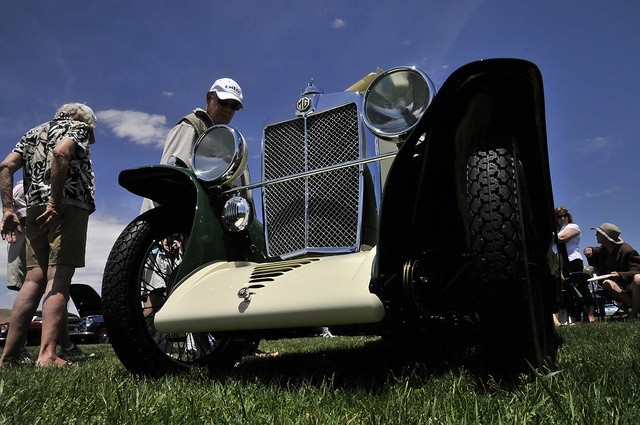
(209, 77), (245, 110)
(591, 223), (624, 244)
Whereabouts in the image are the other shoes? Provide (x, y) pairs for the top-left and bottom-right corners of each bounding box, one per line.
(18, 345), (35, 366)
(57, 344), (102, 361)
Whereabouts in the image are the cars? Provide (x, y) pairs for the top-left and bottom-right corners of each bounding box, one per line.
(0, 310), (82, 344)
(45, 282), (113, 346)
(102, 56), (563, 394)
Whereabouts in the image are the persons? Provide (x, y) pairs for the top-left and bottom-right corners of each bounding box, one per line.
(144, 77), (278, 357)
(0, 103), (97, 368)
(6, 179), (95, 363)
(583, 223), (640, 316)
(552, 207), (596, 326)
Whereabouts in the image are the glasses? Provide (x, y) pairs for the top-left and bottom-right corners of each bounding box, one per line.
(556, 214), (566, 218)
(210, 94), (241, 111)
